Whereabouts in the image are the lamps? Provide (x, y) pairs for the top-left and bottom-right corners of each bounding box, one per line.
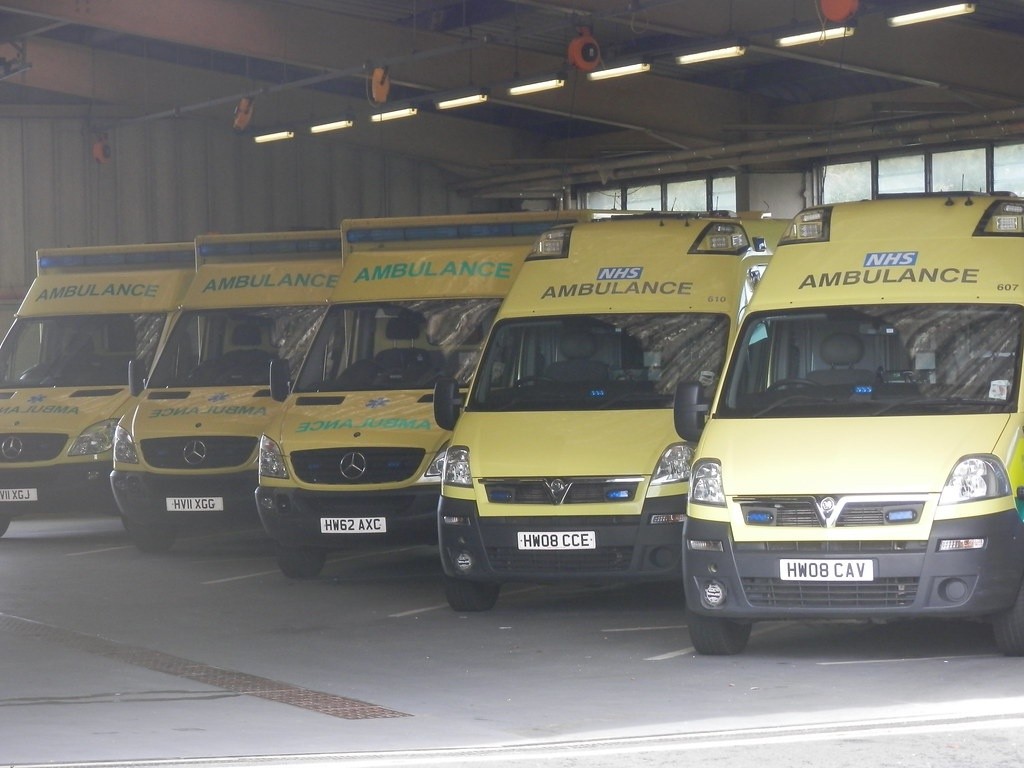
(310, 120), (354, 134)
(438, 94), (488, 109)
(253, 129), (294, 143)
(886, 2), (976, 27)
(774, 26), (855, 48)
(675, 45), (747, 65)
(507, 79), (566, 95)
(369, 107), (417, 122)
(586, 62), (651, 81)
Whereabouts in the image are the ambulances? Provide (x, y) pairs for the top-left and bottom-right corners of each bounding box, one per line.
(254, 205), (774, 579)
(674, 190), (1024, 660)
(1, 243), (201, 538)
(109, 229), (482, 552)
(430, 217), (798, 616)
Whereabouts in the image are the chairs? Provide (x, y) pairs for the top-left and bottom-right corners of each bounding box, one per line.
(372, 317), (438, 379)
(540, 330), (608, 380)
(806, 331), (887, 397)
(63, 332), (100, 367)
(210, 323), (273, 374)
